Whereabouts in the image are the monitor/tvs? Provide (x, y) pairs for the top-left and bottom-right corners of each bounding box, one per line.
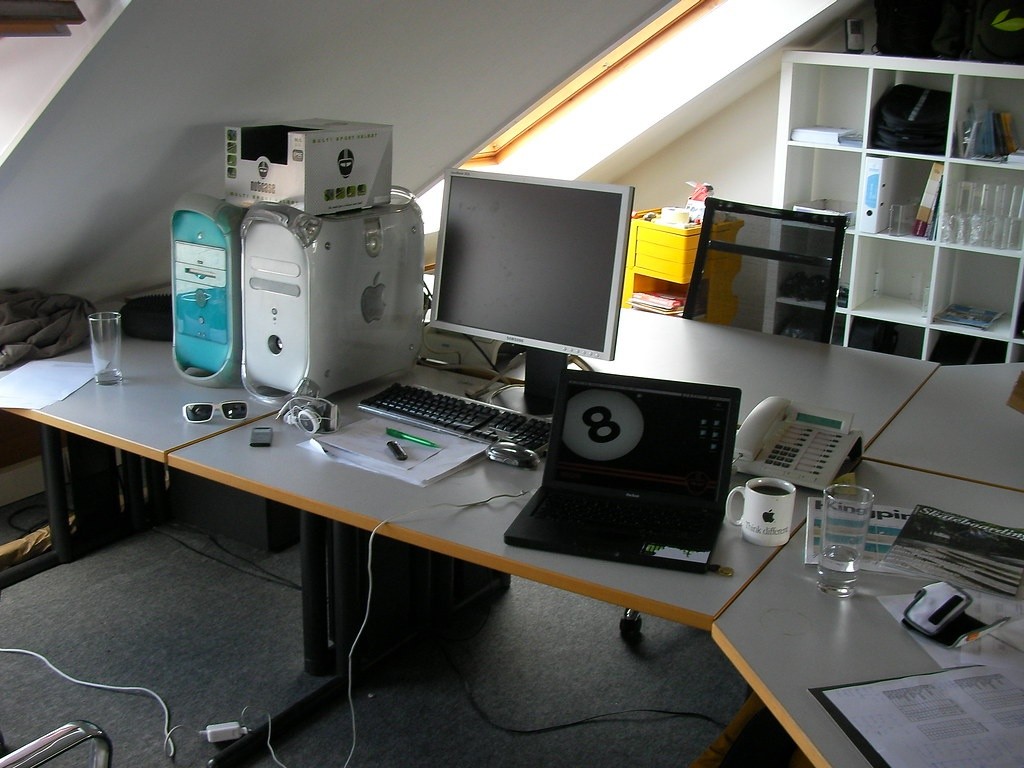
(428, 166), (636, 361)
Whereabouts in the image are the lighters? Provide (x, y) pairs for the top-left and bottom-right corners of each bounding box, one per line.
(386, 440), (408, 460)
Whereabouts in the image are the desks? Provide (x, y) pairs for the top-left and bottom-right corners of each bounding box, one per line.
(0, 310), (1024, 768)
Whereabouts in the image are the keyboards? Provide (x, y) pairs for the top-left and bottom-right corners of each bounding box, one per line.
(355, 382), (554, 458)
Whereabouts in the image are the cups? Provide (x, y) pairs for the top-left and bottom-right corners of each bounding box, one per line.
(88, 312), (124, 386)
(726, 477), (796, 547)
(816, 485), (875, 599)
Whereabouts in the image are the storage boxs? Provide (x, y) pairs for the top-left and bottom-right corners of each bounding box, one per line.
(222, 117), (393, 217)
(621, 209), (744, 326)
(763, 51), (1024, 371)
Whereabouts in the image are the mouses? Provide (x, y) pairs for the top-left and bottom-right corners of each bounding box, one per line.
(486, 442), (541, 470)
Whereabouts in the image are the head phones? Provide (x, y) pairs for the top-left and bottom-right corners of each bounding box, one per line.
(275, 397), (342, 434)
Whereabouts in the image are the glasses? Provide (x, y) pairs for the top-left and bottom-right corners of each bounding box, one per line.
(182, 400), (249, 424)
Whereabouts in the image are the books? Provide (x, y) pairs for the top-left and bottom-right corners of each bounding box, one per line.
(804, 493), (940, 583)
(879, 501), (1024, 598)
(911, 162), (943, 237)
(628, 292), (687, 316)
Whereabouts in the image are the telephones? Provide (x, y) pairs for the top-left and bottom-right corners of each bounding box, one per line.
(733, 396), (862, 492)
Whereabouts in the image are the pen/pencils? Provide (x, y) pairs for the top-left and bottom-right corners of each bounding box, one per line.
(384, 427), (438, 449)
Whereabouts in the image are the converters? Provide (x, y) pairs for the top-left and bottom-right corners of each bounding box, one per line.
(206, 722), (242, 742)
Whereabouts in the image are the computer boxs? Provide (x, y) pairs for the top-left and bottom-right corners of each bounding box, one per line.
(170, 189), (248, 387)
(240, 184), (424, 408)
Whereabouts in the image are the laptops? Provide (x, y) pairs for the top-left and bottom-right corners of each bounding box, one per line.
(503, 368), (741, 575)
